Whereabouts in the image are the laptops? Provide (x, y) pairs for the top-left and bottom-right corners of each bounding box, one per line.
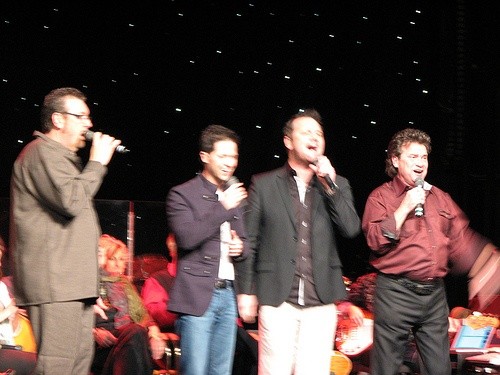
(450, 322), (497, 352)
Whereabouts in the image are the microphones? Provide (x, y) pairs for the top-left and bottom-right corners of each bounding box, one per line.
(86, 131), (130, 153)
(414, 178), (424, 217)
(325, 174), (335, 190)
(226, 177), (248, 208)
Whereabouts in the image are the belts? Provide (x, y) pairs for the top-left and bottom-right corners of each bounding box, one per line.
(216, 278), (234, 289)
(389, 274), (442, 295)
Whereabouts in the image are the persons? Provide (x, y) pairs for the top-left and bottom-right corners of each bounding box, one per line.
(0, 233), (377, 375)
(236, 110), (361, 375)
(165, 123), (248, 375)
(10, 88), (121, 375)
(362, 128), (500, 375)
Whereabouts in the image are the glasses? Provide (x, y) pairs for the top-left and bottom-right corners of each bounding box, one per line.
(61, 112), (92, 120)
